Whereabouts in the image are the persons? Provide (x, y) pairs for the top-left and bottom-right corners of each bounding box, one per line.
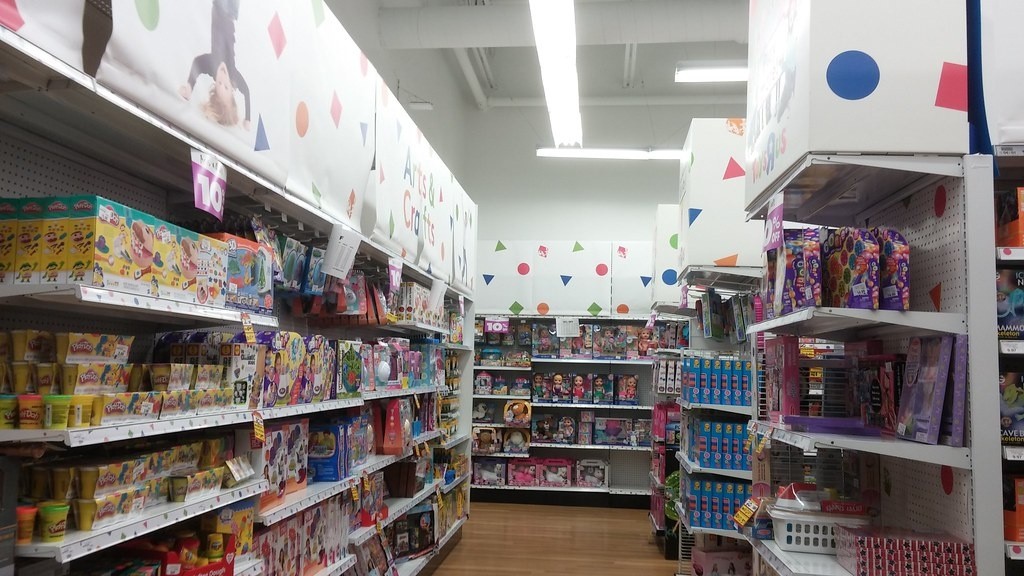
(538, 328), (651, 356)
(178, 0), (251, 132)
(533, 373), (637, 400)
(307, 354), (317, 387)
(273, 352), (283, 389)
(561, 418), (573, 439)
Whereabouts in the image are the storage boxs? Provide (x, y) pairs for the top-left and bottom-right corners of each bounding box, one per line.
(0, 0), (1024, 576)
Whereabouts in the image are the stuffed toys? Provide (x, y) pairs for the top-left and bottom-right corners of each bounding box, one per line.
(478, 463), (604, 487)
(476, 403), (526, 448)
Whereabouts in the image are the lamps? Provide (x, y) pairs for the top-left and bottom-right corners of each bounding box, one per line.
(673, 59), (749, 81)
(534, 146), (683, 157)
(529, 0), (591, 148)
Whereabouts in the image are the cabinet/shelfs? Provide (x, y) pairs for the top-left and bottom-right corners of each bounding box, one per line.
(649, 300), (694, 559)
(531, 314), (682, 495)
(745, 154), (1005, 576)
(471, 313), (530, 491)
(0, 26), (473, 576)
(673, 265), (760, 576)
